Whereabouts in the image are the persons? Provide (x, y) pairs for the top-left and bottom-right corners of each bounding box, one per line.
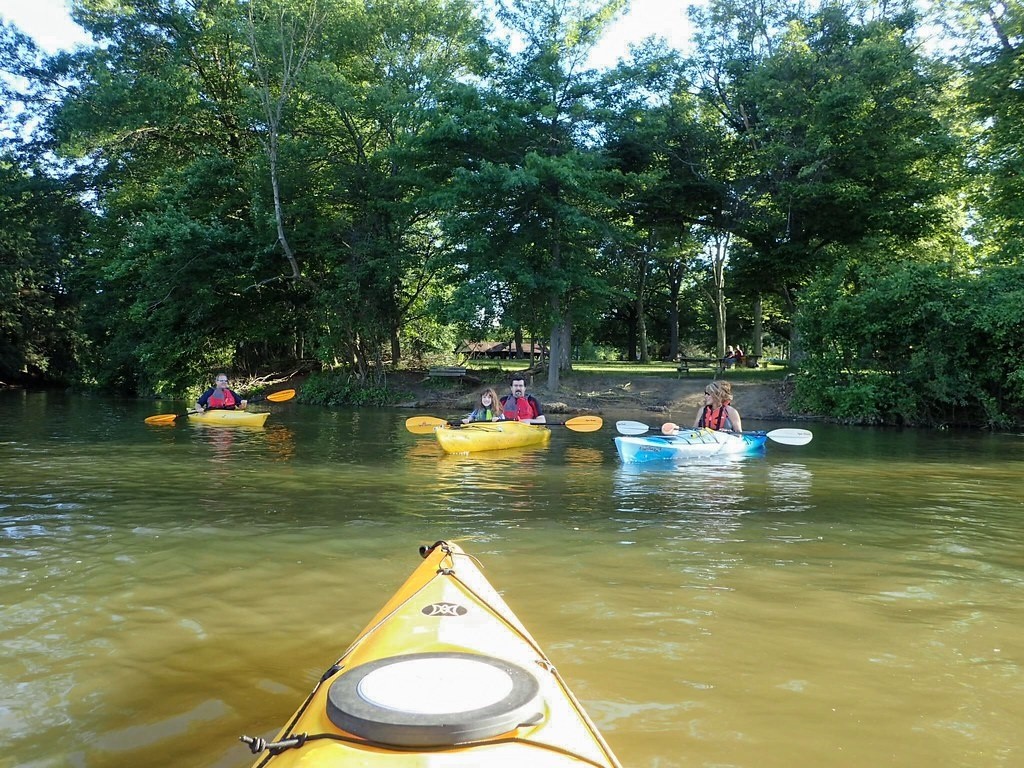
(195, 373), (247, 412)
(693, 381), (742, 438)
(722, 344), (743, 370)
(500, 375), (546, 424)
(462, 388), (505, 424)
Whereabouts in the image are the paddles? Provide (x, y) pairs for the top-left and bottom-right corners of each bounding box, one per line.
(406, 416), (604, 433)
(144, 390), (295, 425)
(615, 420), (813, 446)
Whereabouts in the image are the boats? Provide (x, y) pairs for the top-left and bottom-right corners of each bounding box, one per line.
(613, 423), (769, 465)
(145, 405), (271, 427)
(432, 417), (552, 454)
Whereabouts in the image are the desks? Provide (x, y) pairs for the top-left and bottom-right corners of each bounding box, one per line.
(675, 357), (728, 380)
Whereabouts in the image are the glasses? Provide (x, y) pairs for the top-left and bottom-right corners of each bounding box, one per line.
(216, 379), (228, 382)
(704, 391), (712, 396)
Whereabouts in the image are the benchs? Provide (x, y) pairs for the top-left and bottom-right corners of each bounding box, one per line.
(420, 367), (466, 395)
(725, 355), (762, 368)
